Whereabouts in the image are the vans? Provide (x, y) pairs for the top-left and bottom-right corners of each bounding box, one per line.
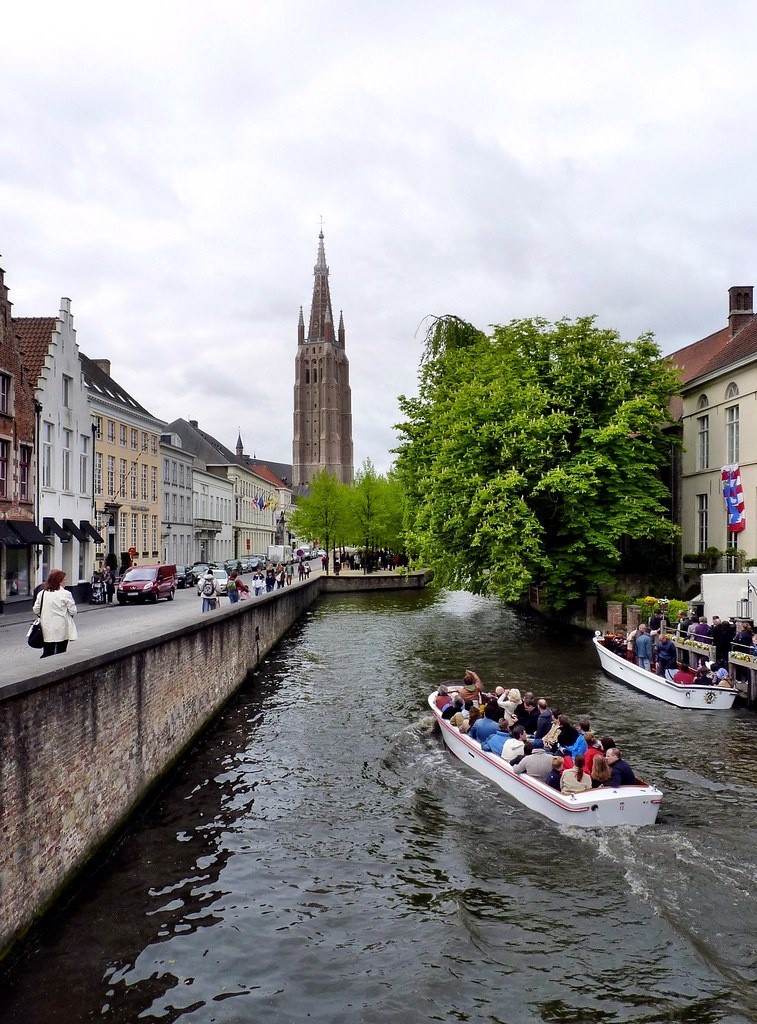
(117, 564), (177, 603)
(9, 562), (50, 597)
(254, 554), (267, 569)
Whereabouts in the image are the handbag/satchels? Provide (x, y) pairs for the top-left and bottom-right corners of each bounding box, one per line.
(275, 571), (283, 582)
(28, 622), (43, 648)
(308, 568), (310, 572)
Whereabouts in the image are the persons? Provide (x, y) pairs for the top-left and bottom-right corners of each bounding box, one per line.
(102, 566), (116, 605)
(252, 560), (294, 596)
(298, 561), (311, 582)
(321, 549), (409, 573)
(32, 570), (77, 658)
(197, 560), (250, 613)
(436, 668), (636, 794)
(626, 608), (757, 688)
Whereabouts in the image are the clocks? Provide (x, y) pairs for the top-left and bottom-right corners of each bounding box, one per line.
(313, 322), (319, 329)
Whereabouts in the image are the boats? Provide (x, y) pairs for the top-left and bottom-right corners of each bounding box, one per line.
(592, 630), (738, 710)
(427, 684), (665, 828)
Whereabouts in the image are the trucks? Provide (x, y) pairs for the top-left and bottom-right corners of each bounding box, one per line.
(300, 544), (313, 561)
(267, 545), (294, 566)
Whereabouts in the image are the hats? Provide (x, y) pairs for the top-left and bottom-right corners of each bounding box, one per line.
(207, 570), (213, 574)
(705, 661), (714, 670)
(533, 739), (544, 749)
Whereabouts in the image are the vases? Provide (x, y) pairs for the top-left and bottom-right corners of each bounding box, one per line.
(659, 603), (668, 608)
(647, 603), (655, 607)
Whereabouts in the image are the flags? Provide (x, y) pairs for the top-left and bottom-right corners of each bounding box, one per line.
(252, 493), (275, 511)
(721, 464), (745, 533)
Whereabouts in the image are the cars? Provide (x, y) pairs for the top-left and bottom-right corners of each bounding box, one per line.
(209, 562), (226, 570)
(312, 550), (326, 559)
(223, 559), (243, 575)
(176, 565), (194, 589)
(238, 559), (252, 573)
(250, 558), (259, 571)
(189, 562), (217, 581)
(291, 553), (298, 563)
(197, 569), (228, 596)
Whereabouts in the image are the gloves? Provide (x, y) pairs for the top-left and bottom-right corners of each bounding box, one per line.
(198, 593), (201, 596)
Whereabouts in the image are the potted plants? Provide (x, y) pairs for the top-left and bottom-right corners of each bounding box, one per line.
(683, 553), (706, 568)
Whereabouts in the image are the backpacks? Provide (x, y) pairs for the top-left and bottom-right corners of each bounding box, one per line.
(226, 578), (238, 596)
(203, 577), (214, 596)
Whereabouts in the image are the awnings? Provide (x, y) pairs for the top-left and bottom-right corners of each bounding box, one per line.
(0, 517), (105, 546)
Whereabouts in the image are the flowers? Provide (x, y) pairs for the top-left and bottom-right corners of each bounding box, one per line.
(645, 596), (657, 602)
(666, 634), (757, 664)
(658, 598), (670, 605)
